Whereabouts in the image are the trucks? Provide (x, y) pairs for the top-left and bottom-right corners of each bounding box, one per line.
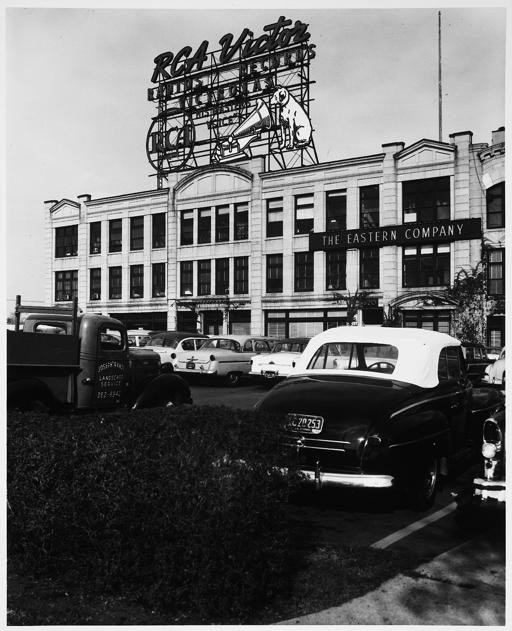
(6, 294), (193, 416)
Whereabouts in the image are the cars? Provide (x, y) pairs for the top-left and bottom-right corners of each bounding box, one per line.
(105, 329), (346, 388)
